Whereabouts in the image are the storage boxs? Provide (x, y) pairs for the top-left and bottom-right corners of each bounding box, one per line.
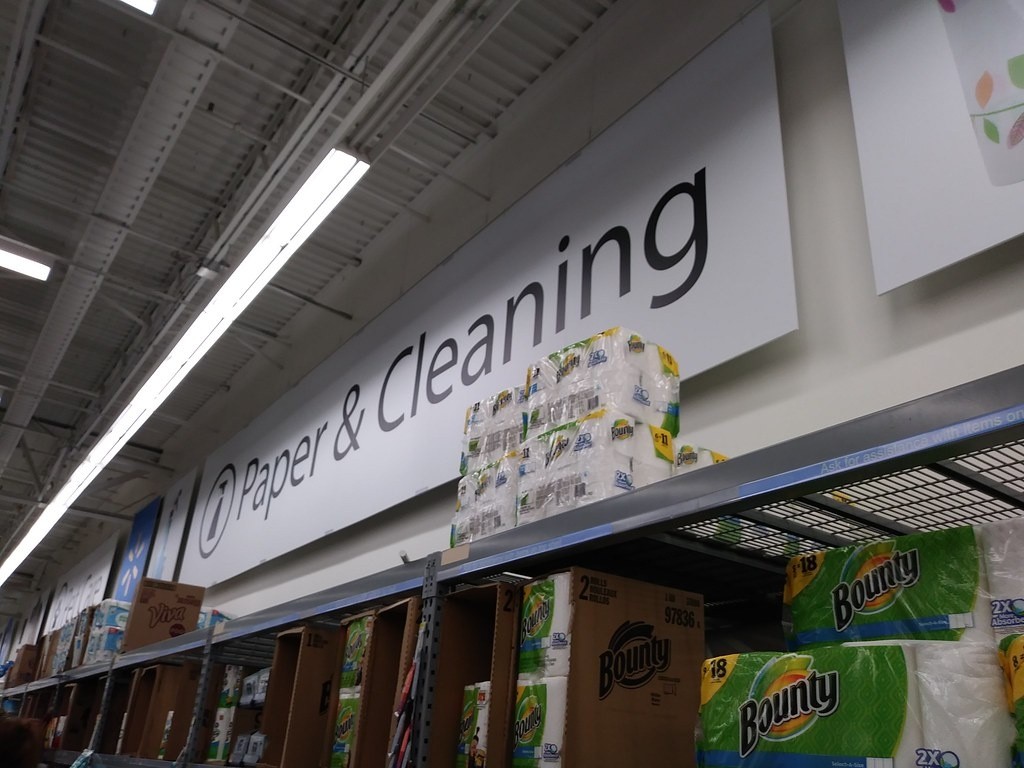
(72, 605), (98, 668)
(58, 683), (90, 749)
(256, 623), (340, 768)
(119, 578), (205, 653)
(5, 643), (37, 688)
(517, 566), (706, 768)
(43, 628), (63, 677)
(323, 607), (377, 768)
(351, 595), (422, 768)
(439, 581), (521, 768)
(119, 664), (224, 763)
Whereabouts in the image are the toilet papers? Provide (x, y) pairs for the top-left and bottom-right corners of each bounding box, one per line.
(1, 516), (1023, 768)
(447, 325), (729, 550)
